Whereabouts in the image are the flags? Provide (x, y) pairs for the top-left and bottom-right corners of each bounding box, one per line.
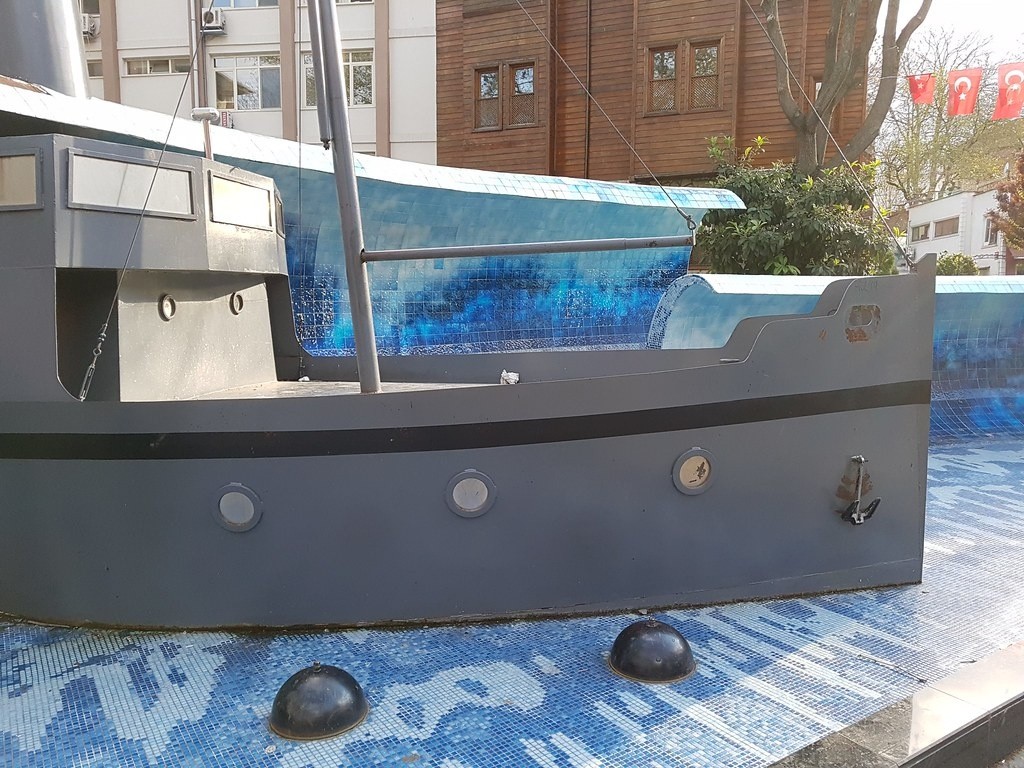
(948, 68), (982, 116)
(908, 73), (936, 105)
(990, 62), (1024, 120)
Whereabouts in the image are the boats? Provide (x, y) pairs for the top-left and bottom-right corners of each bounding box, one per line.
(0, 0), (935, 627)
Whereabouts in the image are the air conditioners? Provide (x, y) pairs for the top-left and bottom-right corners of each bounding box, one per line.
(82, 12), (95, 34)
(202, 7), (225, 29)
